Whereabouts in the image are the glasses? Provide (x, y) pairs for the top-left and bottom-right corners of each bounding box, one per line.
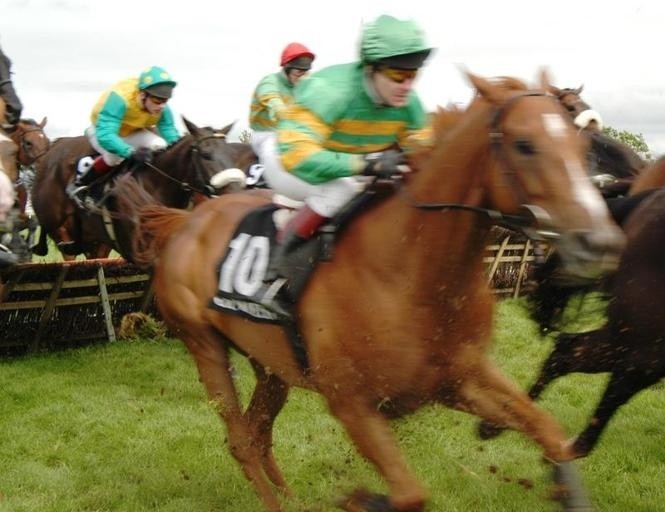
(378, 66), (417, 83)
(289, 68), (304, 77)
(150, 97), (168, 104)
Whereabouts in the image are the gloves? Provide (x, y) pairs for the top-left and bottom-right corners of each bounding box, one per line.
(132, 147), (153, 163)
(362, 150), (412, 180)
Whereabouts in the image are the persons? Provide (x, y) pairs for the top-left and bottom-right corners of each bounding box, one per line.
(73, 66), (182, 201)
(258, 13), (436, 298)
(248, 42), (316, 160)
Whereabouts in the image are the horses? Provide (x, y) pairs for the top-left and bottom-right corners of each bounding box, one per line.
(0, 43), (259, 266)
(548, 84), (665, 197)
(476, 186), (665, 467)
(92, 68), (630, 511)
(22, 114), (247, 264)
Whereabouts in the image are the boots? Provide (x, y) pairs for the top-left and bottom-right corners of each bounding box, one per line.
(65, 167), (100, 211)
(244, 231), (307, 322)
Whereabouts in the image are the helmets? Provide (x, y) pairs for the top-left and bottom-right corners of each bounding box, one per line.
(144, 84), (172, 99)
(380, 54), (424, 69)
(285, 55), (313, 70)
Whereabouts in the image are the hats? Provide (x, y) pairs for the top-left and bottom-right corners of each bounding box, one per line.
(280, 42), (314, 66)
(361, 15), (434, 63)
(139, 66), (176, 90)
